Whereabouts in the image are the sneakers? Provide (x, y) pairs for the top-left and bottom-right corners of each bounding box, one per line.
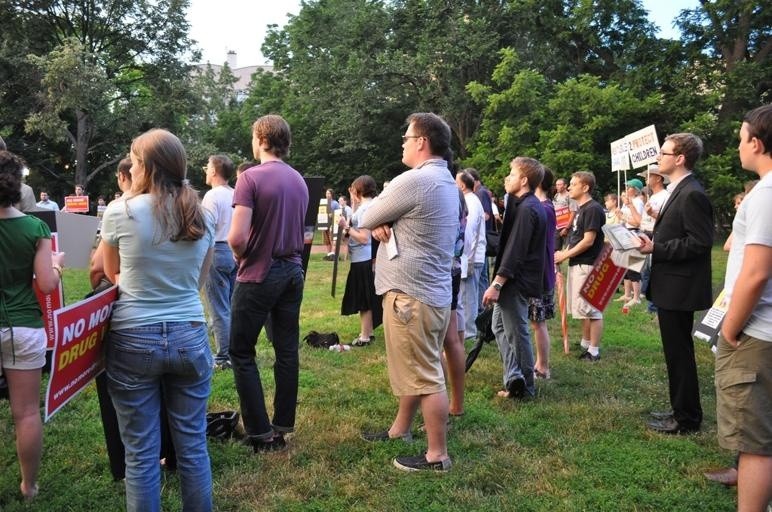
(578, 348), (600, 363)
(346, 336), (376, 348)
(359, 429), (413, 444)
(391, 451), (452, 476)
(613, 295), (643, 308)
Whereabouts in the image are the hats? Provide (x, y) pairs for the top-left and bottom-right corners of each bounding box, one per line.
(623, 164), (671, 193)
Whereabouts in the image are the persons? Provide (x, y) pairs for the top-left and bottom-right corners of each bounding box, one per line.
(624, 131), (713, 434)
(324, 176), (391, 347)
(355, 111), (461, 474)
(454, 157), (668, 400)
(723, 181), (759, 251)
(0, 149), (62, 499)
(218, 114), (311, 454)
(702, 107), (771, 512)
(100, 128), (222, 512)
(17, 159), (177, 486)
(201, 155), (257, 367)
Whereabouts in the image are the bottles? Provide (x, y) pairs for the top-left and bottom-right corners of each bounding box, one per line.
(328, 344), (352, 354)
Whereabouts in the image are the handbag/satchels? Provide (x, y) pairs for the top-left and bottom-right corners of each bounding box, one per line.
(302, 330), (341, 350)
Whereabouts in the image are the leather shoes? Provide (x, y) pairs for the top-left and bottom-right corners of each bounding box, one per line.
(702, 466), (739, 484)
(648, 410), (693, 433)
(238, 430), (287, 455)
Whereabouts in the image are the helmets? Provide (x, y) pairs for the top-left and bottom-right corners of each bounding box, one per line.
(205, 408), (241, 441)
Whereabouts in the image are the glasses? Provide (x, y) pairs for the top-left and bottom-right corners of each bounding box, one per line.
(401, 135), (427, 144)
(659, 150), (676, 157)
